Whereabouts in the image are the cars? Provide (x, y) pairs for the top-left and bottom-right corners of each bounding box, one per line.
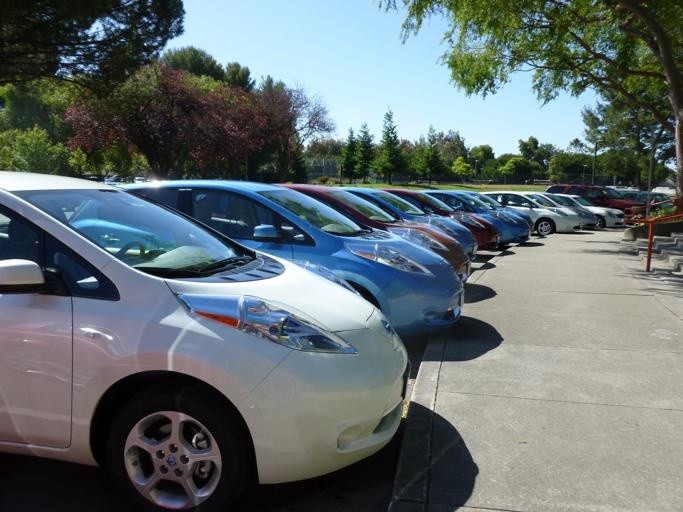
(559, 194), (625, 228)
(537, 191), (600, 231)
(422, 190), (532, 245)
(0, 172), (411, 509)
(116, 181), (463, 340)
(291, 184), (472, 284)
(335, 186), (479, 262)
(611, 185), (675, 212)
(478, 191), (582, 237)
(382, 189), (502, 251)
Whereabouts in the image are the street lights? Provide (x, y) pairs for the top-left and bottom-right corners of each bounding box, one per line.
(582, 165), (589, 185)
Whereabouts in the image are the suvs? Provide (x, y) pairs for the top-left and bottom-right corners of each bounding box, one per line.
(544, 184), (646, 226)
